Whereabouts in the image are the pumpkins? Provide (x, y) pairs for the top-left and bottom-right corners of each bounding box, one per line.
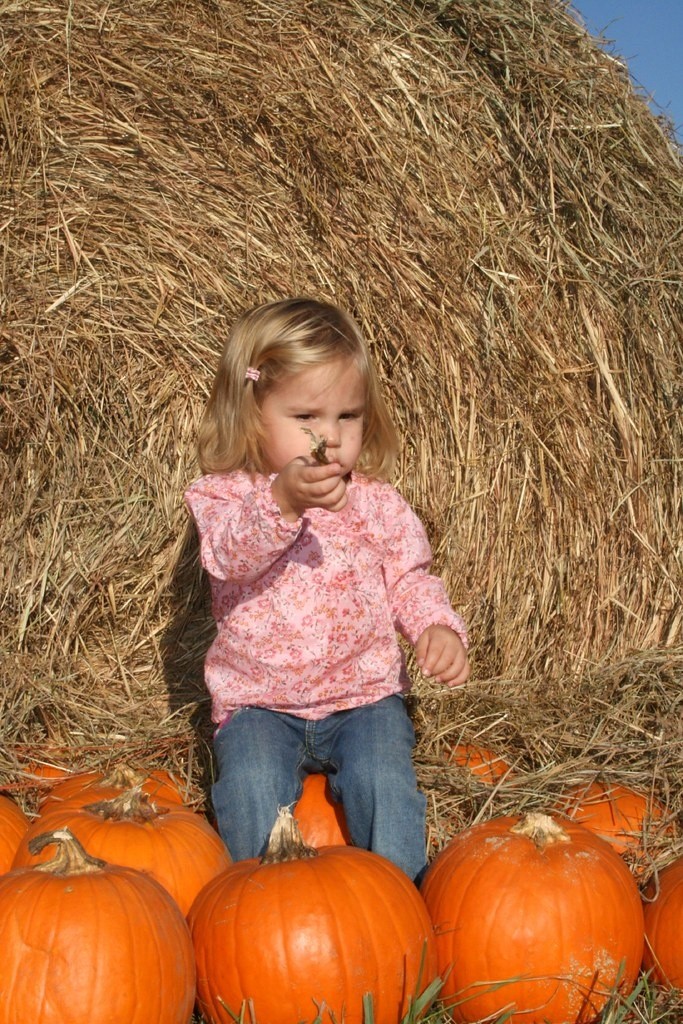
(0, 743), (683, 1024)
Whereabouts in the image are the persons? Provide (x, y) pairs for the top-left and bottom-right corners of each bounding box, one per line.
(186, 299), (471, 886)
